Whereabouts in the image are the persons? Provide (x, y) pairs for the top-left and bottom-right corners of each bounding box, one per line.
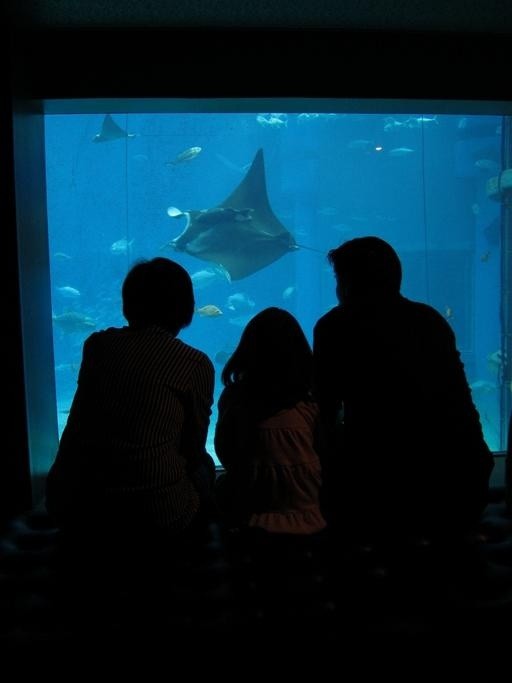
(212, 305), (337, 682)
(309, 233), (499, 682)
(42, 254), (218, 683)
